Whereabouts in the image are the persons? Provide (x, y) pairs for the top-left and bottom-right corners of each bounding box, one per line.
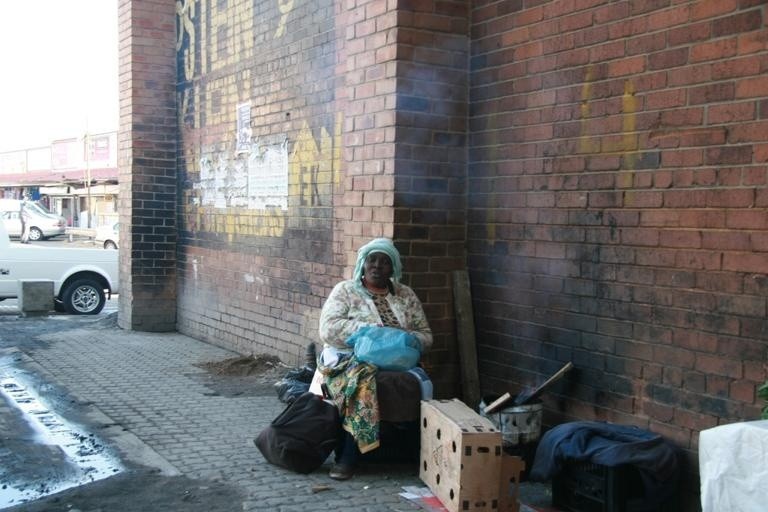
(18, 194), (33, 245)
(308, 236), (435, 481)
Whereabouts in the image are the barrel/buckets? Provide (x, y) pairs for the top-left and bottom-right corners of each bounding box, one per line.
(479, 393), (544, 447)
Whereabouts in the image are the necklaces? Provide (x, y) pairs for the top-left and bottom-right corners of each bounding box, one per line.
(365, 284), (389, 295)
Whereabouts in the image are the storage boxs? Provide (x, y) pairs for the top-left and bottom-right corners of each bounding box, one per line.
(419, 397), (504, 512)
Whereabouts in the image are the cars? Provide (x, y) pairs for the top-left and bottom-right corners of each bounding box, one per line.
(0, 241), (128, 315)
(1, 209), (66, 241)
(92, 221), (130, 250)
(2, 199), (67, 242)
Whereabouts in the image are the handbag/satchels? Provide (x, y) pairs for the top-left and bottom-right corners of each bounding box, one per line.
(254, 384), (340, 473)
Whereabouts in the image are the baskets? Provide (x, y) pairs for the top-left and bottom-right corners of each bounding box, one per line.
(552, 461), (640, 512)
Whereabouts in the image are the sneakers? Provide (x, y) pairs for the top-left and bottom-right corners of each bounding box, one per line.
(329, 459), (353, 480)
